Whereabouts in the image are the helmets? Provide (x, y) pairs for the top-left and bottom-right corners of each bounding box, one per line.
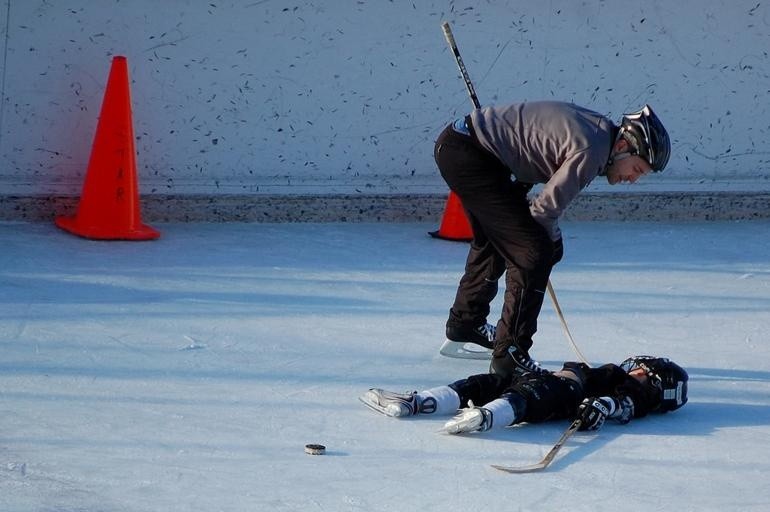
(619, 103), (672, 173)
(619, 352), (690, 415)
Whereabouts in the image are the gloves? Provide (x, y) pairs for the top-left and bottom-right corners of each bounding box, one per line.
(567, 395), (635, 434)
(553, 237), (564, 265)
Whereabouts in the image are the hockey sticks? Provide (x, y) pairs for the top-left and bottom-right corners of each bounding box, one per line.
(490, 417), (582, 472)
(442, 20), (594, 369)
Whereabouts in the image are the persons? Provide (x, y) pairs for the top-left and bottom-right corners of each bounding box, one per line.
(434, 101), (671, 376)
(357, 354), (689, 434)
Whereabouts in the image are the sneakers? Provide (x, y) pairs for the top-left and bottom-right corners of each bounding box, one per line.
(445, 307), (496, 351)
(365, 386), (422, 419)
(492, 343), (550, 377)
(445, 404), (494, 435)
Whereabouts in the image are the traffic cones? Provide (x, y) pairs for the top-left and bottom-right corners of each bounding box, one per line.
(49, 50), (159, 245)
(424, 188), (477, 246)
